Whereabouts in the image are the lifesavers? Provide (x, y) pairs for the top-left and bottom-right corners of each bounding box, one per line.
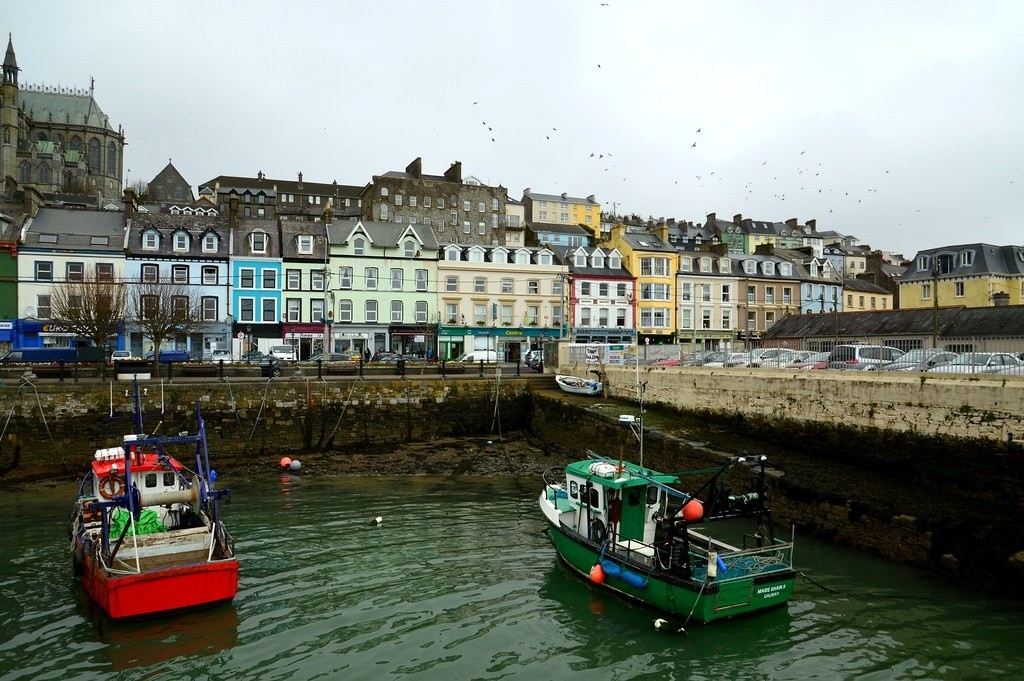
(99, 477), (126, 501)
(591, 519), (606, 543)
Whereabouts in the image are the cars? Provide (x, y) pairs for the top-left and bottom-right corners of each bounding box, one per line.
(301, 353), (358, 375)
(379, 354), (427, 363)
(786, 351), (831, 370)
(524, 349), (544, 372)
(212, 349), (233, 364)
(370, 352), (393, 364)
(927, 352), (1024, 376)
(345, 350), (363, 361)
(878, 348), (961, 373)
(110, 350), (137, 365)
(650, 346), (795, 369)
(760, 351), (820, 370)
(188, 352), (202, 364)
(241, 344), (296, 378)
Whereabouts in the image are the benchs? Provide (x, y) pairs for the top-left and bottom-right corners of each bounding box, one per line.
(181, 366), (217, 375)
(437, 364), (466, 374)
(327, 366), (357, 376)
(31, 367), (74, 379)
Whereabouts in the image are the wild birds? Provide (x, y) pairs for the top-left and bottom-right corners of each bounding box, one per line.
(470, 97), (1016, 222)
(598, 2), (609, 7)
(596, 63), (602, 69)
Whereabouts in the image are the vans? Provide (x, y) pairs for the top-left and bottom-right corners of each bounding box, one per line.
(1, 347), (78, 365)
(446, 350), (497, 364)
(146, 351), (191, 366)
(828, 345), (907, 371)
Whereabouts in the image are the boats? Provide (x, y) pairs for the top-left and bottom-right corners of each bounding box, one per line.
(537, 449), (796, 626)
(556, 374), (602, 396)
(68, 417), (239, 619)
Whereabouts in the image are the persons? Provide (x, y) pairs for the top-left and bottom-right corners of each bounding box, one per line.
(364, 348), (372, 363)
(427, 347), (430, 362)
(390, 350), (393, 357)
(376, 352), (380, 363)
